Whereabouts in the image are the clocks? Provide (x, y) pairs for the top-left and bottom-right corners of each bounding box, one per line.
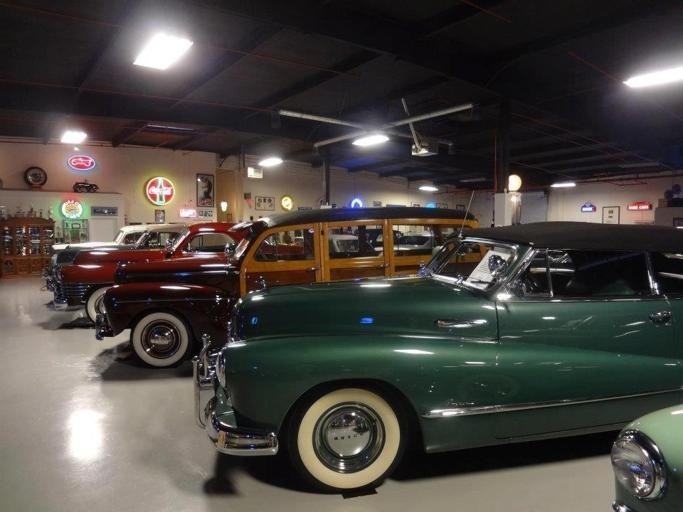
(25, 166), (48, 187)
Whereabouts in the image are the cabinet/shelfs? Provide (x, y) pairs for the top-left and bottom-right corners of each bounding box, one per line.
(0, 216), (57, 277)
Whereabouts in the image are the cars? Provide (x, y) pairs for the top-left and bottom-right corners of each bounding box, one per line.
(189, 219), (683, 495)
(40, 221), (453, 329)
(612, 404), (682, 512)
(95, 205), (494, 372)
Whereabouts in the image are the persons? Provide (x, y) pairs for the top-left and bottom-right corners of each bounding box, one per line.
(198, 176), (212, 202)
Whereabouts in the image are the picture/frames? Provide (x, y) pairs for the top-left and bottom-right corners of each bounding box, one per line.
(197, 174), (216, 207)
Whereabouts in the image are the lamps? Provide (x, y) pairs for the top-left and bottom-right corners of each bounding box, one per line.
(508, 174), (523, 227)
